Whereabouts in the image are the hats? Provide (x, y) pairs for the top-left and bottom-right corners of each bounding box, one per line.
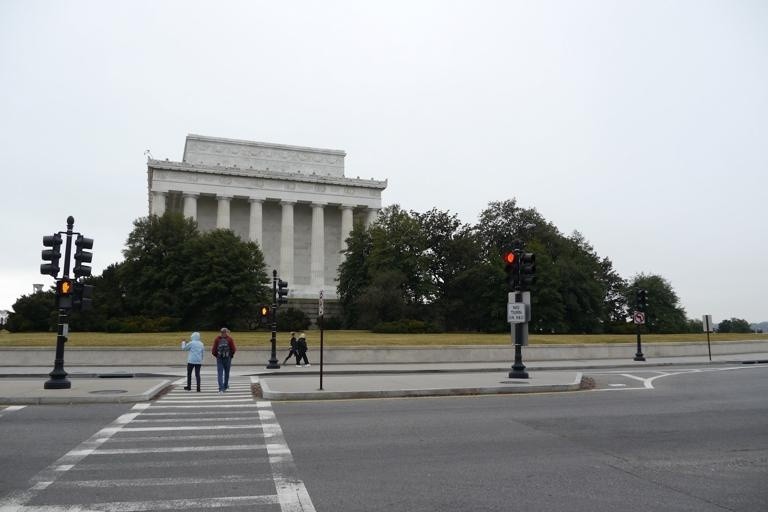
(220, 328), (227, 332)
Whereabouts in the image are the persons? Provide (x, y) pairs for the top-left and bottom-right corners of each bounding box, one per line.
(211, 327), (236, 395)
(283, 332), (299, 364)
(181, 331), (204, 393)
(296, 333), (311, 368)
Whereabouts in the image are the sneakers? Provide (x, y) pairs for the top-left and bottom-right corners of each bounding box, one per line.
(184, 386), (191, 390)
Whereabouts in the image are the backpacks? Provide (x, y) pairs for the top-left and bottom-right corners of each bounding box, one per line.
(217, 338), (231, 359)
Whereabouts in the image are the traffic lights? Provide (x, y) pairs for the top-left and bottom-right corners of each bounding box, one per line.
(56, 279), (96, 314)
(277, 280), (289, 306)
(257, 305), (270, 324)
(40, 233), (62, 277)
(502, 251), (537, 288)
(636, 288), (649, 312)
(73, 234), (93, 278)
